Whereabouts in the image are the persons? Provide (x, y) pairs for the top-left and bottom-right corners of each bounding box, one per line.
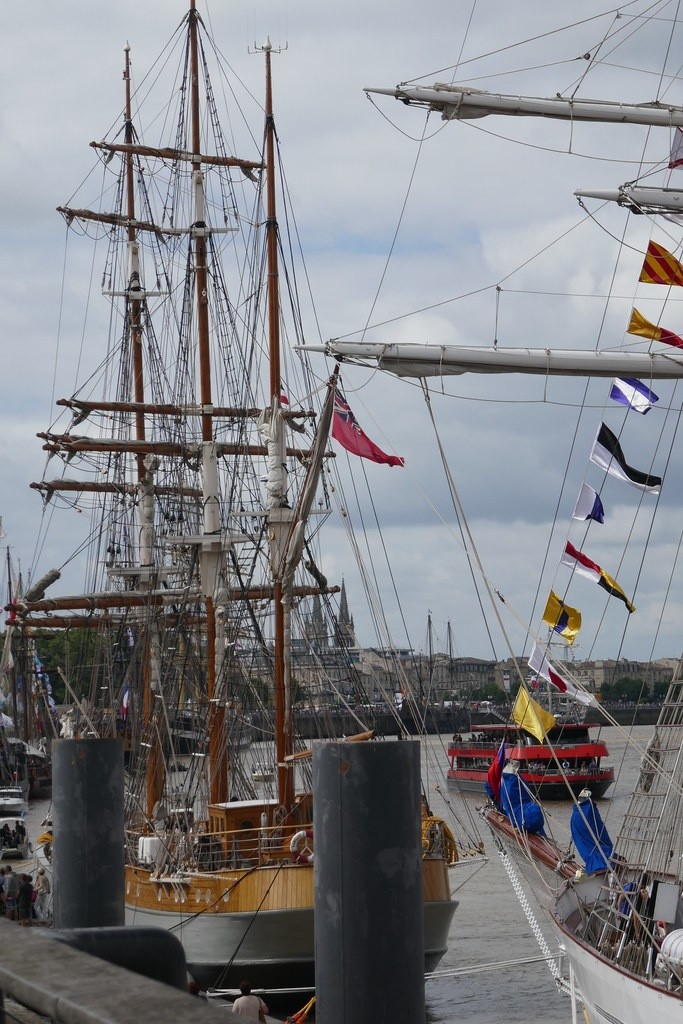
(617, 873), (650, 943)
(17, 873), (34, 925)
(0, 868), (5, 916)
(2, 866), (20, 920)
(0, 824), (25, 848)
(455, 731), (516, 742)
(34, 868), (50, 920)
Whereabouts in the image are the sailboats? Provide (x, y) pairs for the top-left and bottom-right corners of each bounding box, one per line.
(0, 2), (462, 1017)
(291, 0), (682, 1024)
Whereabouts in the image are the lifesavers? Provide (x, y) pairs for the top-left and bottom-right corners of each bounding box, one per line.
(288, 829), (314, 864)
(654, 921), (666, 948)
(562, 761), (570, 769)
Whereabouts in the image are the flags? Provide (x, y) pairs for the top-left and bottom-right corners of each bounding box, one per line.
(544, 591), (583, 645)
(529, 645), (591, 707)
(572, 800), (615, 871)
(591, 422), (661, 497)
(561, 541), (636, 613)
(609, 376), (658, 415)
(669, 127), (683, 169)
(512, 687), (555, 741)
(332, 388), (404, 466)
(501, 770), (542, 834)
(489, 740), (506, 798)
(573, 483), (604, 524)
(628, 308), (683, 349)
(639, 241), (683, 286)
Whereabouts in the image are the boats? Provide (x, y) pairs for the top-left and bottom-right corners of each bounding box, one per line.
(447, 627), (615, 802)
(0, 785), (29, 817)
(0, 817), (33, 861)
(251, 763), (276, 783)
(166, 688), (254, 756)
